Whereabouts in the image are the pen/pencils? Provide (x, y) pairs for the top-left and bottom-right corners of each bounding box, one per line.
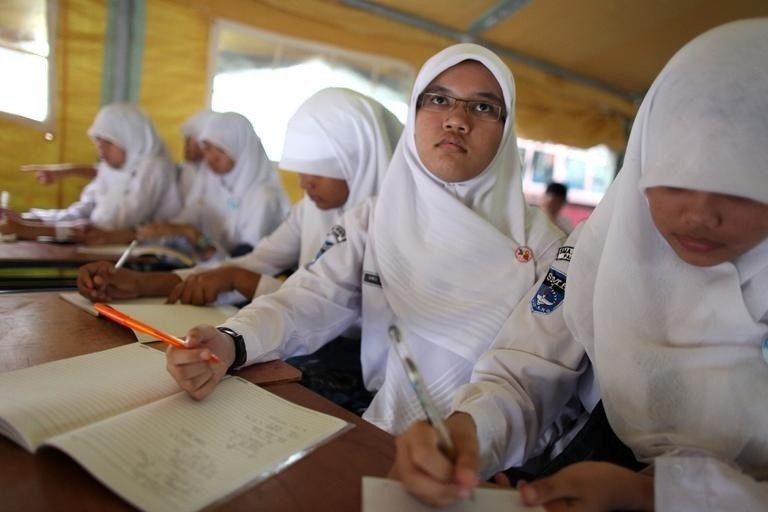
(0, 191), (9, 225)
(94, 302), (222, 365)
(97, 239), (138, 297)
(389, 324), (477, 505)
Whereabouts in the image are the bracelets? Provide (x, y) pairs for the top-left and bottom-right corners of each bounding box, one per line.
(217, 327), (247, 369)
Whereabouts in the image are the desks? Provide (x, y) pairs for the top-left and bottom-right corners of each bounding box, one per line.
(2, 234), (304, 385)
(2, 381), (538, 511)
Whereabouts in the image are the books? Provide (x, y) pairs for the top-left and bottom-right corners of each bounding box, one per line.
(61, 289), (240, 345)
(360, 475), (547, 512)
(1, 343), (355, 511)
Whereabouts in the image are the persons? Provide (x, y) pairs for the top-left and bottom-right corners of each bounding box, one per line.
(72, 87), (404, 418)
(525, 182), (573, 237)
(165, 43), (569, 489)
(395, 15), (767, 512)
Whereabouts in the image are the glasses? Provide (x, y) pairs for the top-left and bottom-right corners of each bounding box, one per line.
(417, 92), (506, 122)
